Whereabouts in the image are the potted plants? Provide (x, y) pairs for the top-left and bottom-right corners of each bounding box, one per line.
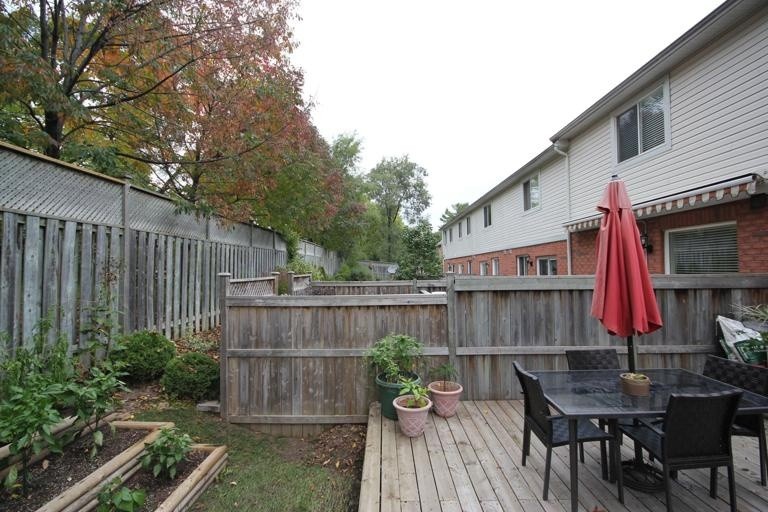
(361, 332), (430, 421)
(393, 373), (433, 438)
(428, 362), (463, 417)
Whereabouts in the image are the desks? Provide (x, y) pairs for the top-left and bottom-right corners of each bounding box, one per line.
(529, 368), (767, 512)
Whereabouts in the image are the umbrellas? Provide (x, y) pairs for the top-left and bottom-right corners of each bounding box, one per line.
(585, 173), (666, 466)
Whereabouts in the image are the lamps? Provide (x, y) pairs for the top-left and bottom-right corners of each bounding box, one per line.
(640, 232), (652, 253)
(527, 256), (533, 266)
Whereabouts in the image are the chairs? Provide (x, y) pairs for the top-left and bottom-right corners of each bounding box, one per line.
(700, 354), (768, 499)
(514, 361), (615, 500)
(565, 349), (621, 463)
(615, 388), (744, 512)
